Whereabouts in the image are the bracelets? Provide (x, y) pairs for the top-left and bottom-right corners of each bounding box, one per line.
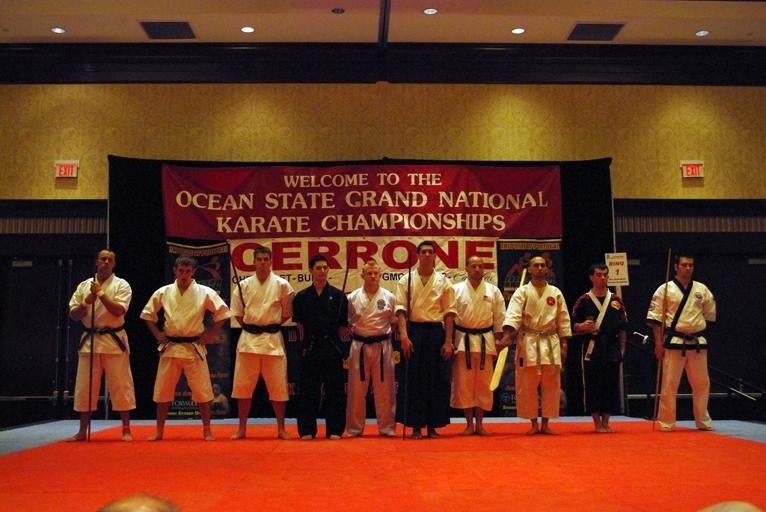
(81, 299), (91, 309)
(94, 289), (105, 298)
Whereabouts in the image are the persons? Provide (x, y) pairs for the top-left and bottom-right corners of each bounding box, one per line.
(290, 253), (352, 441)
(199, 255), (220, 283)
(645, 251), (718, 433)
(542, 250), (557, 284)
(229, 246), (295, 441)
(139, 253), (232, 441)
(66, 250), (137, 442)
(505, 250), (533, 282)
(694, 498), (762, 512)
(98, 493), (179, 512)
(346, 259), (399, 439)
(211, 381), (230, 416)
(442, 254), (507, 437)
(571, 262), (629, 434)
(394, 240), (457, 440)
(497, 255), (572, 437)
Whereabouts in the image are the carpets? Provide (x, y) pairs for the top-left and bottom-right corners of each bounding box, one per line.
(0, 420), (766, 512)
